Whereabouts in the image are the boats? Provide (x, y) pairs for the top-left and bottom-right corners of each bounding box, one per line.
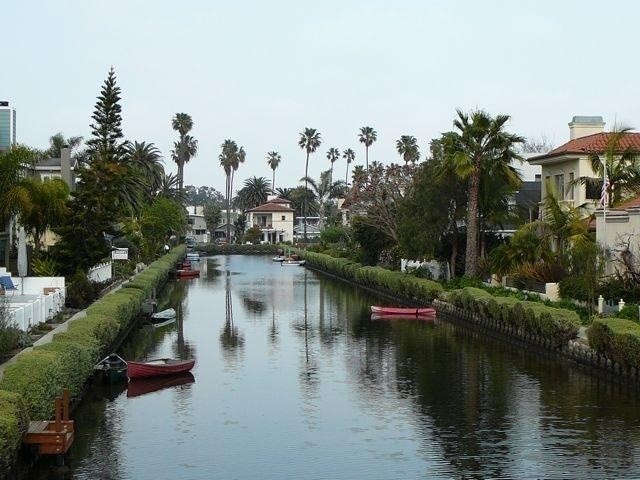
(96, 353), (195, 385)
(178, 270), (200, 276)
(371, 314), (436, 320)
(151, 308), (176, 321)
(272, 255), (305, 266)
(371, 305), (436, 314)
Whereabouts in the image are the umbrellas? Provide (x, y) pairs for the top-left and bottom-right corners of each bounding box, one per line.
(16, 225), (28, 296)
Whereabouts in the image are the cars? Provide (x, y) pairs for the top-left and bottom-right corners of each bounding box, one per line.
(187, 238), (197, 248)
(215, 238), (227, 245)
